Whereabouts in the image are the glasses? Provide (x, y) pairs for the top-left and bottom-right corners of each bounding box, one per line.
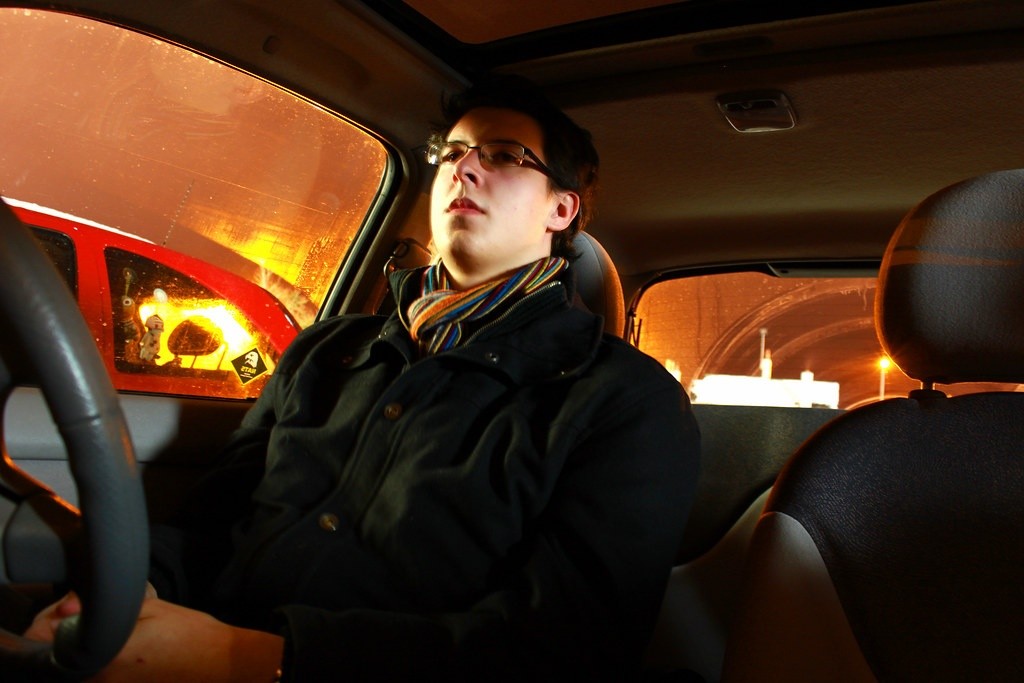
(426, 139), (571, 191)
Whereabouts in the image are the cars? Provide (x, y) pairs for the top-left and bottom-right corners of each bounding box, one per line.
(5, 199), (304, 402)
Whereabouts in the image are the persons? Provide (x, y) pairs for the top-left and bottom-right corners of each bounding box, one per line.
(21, 71), (701, 683)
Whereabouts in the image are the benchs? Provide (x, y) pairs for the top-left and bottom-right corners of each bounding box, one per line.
(637, 400), (846, 683)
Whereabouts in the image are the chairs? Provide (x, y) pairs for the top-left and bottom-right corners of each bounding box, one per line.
(568, 228), (626, 338)
(741, 165), (1024, 683)
